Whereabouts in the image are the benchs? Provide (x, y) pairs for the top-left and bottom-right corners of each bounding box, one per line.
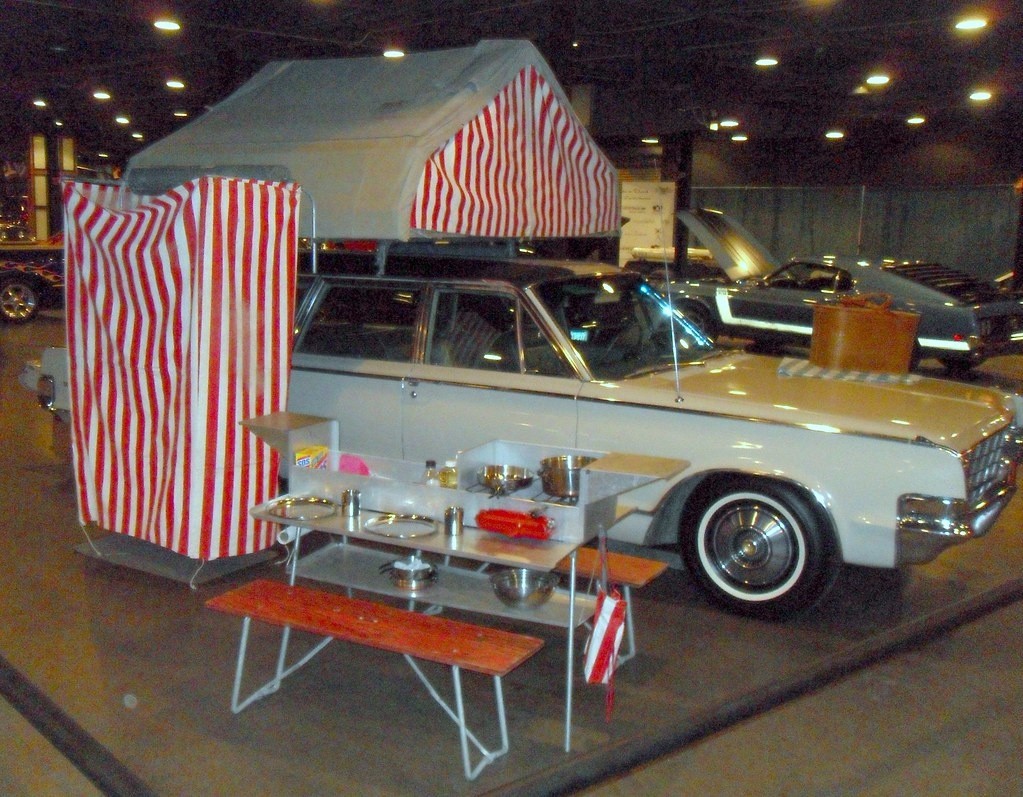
(555, 548), (669, 671)
(205, 577), (547, 779)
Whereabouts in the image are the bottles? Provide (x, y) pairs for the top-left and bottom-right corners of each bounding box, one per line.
(422, 460), (441, 487)
(439, 461), (458, 489)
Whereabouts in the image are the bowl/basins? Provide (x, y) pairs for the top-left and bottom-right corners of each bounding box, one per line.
(489, 569), (561, 609)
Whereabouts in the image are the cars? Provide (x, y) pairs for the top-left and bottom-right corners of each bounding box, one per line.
(649, 206), (1023, 376)
(0, 254), (65, 326)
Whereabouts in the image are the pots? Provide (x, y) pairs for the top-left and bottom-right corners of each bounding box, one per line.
(476, 465), (534, 495)
(377, 557), (438, 591)
(536, 455), (597, 497)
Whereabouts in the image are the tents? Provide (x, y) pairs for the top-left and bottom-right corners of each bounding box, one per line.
(123, 40), (625, 247)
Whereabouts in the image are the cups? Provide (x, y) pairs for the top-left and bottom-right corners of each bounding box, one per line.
(341, 490), (361, 516)
(445, 506), (463, 536)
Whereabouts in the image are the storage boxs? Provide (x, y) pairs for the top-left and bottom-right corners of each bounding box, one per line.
(810, 290), (919, 375)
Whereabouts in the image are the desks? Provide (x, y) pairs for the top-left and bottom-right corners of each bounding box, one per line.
(251, 494), (638, 754)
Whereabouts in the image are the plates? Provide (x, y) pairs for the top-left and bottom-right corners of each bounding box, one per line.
(364, 514), (438, 539)
(265, 495), (337, 521)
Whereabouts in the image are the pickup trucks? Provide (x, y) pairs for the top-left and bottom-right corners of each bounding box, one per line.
(19, 251), (1023, 624)
